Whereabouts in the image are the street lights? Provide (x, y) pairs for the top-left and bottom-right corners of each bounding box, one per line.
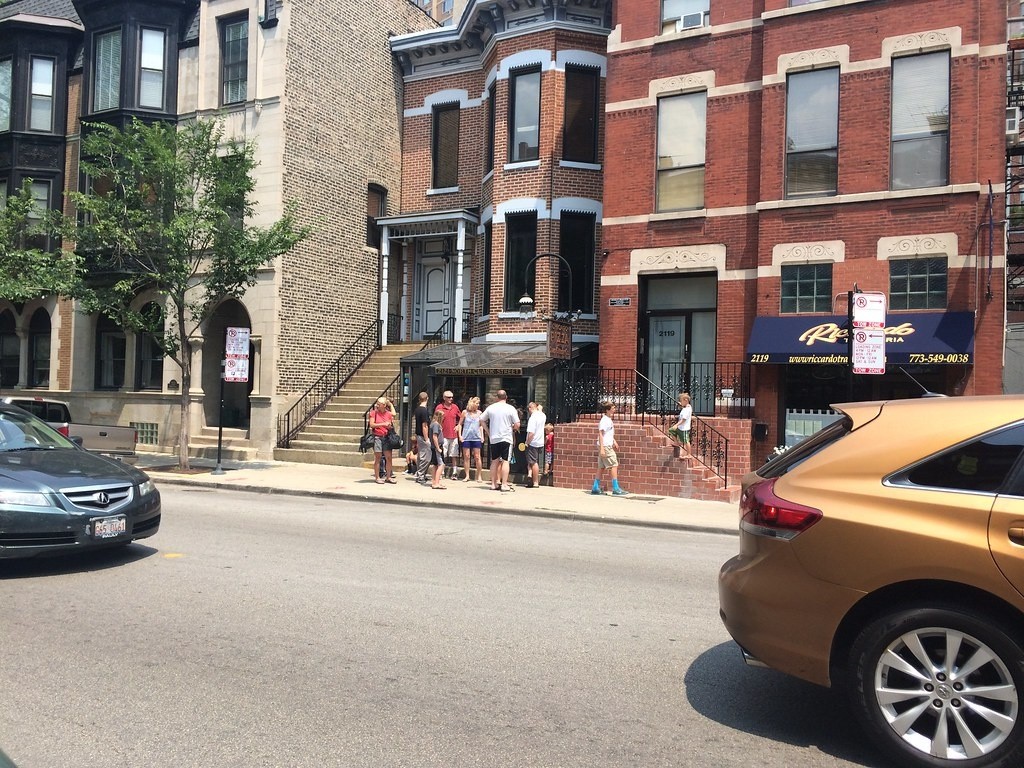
(518, 252), (573, 420)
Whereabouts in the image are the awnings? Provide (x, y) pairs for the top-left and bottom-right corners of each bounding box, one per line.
(745, 311), (976, 366)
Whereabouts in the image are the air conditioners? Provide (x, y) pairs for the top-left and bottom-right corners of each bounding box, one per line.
(1005, 106), (1019, 148)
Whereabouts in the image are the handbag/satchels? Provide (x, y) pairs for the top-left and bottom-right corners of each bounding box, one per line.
(361, 433), (374, 448)
(386, 429), (404, 449)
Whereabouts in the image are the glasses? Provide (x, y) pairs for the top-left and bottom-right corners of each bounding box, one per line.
(445, 397), (453, 399)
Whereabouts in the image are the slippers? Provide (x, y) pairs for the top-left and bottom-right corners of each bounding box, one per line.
(524, 484), (539, 488)
(489, 484), (500, 490)
(501, 487), (514, 492)
(667, 444), (679, 447)
(679, 456), (692, 460)
(527, 475), (533, 488)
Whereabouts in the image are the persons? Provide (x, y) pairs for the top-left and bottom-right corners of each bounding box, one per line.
(524, 401), (554, 488)
(590, 402), (628, 496)
(668, 393), (693, 460)
(369, 397), (397, 484)
(402, 390), (523, 492)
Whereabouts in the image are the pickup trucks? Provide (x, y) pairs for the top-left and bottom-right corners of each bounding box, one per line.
(0, 396), (138, 467)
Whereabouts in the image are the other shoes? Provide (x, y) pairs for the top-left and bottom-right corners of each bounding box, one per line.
(385, 478), (397, 484)
(543, 473), (549, 478)
(432, 484), (447, 489)
(375, 479), (384, 483)
(402, 469), (412, 474)
(411, 470), (416, 474)
(451, 474), (457, 479)
(415, 478), (428, 483)
(440, 475), (442, 479)
(477, 478), (482, 483)
(462, 477), (470, 481)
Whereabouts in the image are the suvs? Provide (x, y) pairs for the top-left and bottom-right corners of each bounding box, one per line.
(716, 392), (1024, 768)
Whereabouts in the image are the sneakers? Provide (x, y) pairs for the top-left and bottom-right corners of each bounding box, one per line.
(612, 488), (628, 496)
(591, 489), (607, 495)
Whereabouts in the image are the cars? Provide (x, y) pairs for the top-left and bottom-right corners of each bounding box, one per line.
(0, 400), (160, 563)
